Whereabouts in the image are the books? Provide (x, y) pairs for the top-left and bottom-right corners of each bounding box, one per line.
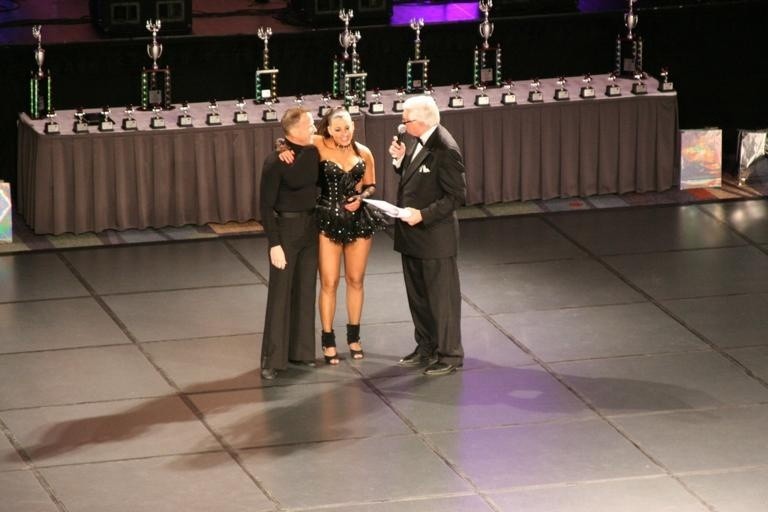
(83, 113), (99, 125)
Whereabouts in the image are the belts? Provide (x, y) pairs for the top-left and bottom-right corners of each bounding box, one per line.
(274, 207), (317, 221)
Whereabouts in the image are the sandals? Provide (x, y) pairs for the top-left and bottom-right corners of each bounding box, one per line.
(324, 347), (341, 365)
(347, 340), (363, 359)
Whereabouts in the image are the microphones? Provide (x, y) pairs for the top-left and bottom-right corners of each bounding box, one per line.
(391, 124), (407, 159)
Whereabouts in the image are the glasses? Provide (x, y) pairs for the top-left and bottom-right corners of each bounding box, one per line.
(401, 120), (415, 126)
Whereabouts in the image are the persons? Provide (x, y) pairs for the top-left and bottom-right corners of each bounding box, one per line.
(389, 95), (467, 375)
(274, 110), (376, 364)
(260, 108), (363, 380)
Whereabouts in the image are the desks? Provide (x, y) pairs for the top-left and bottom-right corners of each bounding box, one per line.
(14, 92), (365, 236)
(359, 72), (682, 209)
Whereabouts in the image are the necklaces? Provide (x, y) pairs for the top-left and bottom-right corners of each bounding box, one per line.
(334, 141), (351, 149)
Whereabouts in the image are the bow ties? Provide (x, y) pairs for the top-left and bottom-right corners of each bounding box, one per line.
(415, 135), (423, 144)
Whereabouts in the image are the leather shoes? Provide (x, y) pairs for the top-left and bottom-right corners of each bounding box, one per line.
(290, 356), (316, 367)
(261, 367), (276, 379)
(422, 359), (464, 376)
(399, 345), (438, 366)
